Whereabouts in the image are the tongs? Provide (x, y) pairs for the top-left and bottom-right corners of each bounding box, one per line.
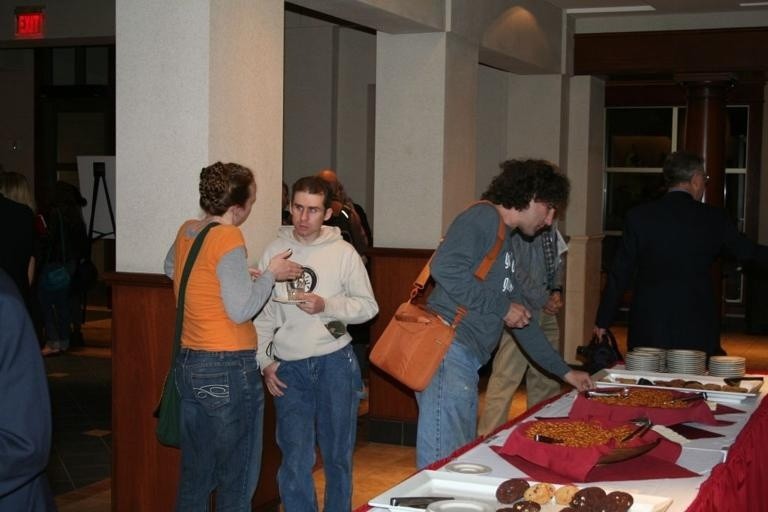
(535, 434), (562, 444)
(724, 376), (765, 386)
(585, 387), (629, 398)
(390, 496), (455, 508)
(618, 417), (652, 443)
(660, 390), (707, 406)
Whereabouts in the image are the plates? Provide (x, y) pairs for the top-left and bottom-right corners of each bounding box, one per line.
(445, 462), (492, 476)
(366, 467), (676, 512)
(516, 417), (663, 465)
(272, 295), (306, 305)
(624, 345), (748, 378)
(588, 367), (764, 405)
(426, 499), (489, 511)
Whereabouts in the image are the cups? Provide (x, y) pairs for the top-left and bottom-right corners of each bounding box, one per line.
(286, 272), (306, 300)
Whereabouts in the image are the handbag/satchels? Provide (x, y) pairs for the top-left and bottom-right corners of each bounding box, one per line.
(368, 303), (456, 393)
(576, 325), (624, 374)
(152, 366), (180, 449)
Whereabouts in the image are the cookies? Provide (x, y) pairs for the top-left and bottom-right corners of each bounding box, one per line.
(495, 477), (634, 512)
(615, 377), (748, 392)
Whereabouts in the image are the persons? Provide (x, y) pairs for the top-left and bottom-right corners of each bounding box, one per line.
(412, 157), (598, 474)
(316, 169), (369, 253)
(1, 164), (92, 512)
(591, 149), (768, 368)
(161, 161), (306, 510)
(281, 181), (295, 225)
(245, 175), (381, 510)
(473, 217), (568, 439)
